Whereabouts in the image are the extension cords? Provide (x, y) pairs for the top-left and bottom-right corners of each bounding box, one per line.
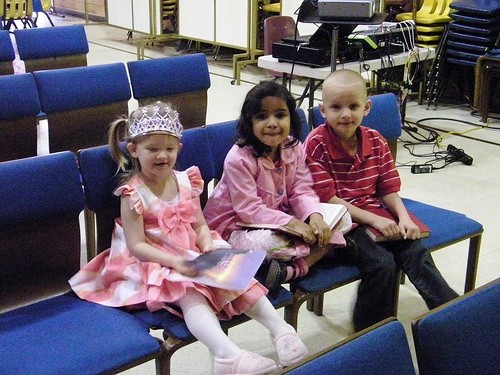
(447, 145), (473, 165)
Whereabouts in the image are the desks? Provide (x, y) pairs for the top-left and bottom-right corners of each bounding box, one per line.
(258, 45), (435, 121)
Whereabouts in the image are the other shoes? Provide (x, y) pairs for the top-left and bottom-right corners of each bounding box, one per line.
(214, 349), (276, 375)
(269, 323), (310, 367)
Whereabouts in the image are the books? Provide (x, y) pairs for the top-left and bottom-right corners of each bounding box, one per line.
(359, 207), (432, 243)
(236, 201), (349, 244)
(170, 246), (266, 294)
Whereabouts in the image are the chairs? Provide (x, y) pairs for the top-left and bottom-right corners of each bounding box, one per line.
(0, 0), (500, 375)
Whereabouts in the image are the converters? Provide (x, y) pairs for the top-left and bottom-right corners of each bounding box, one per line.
(411, 164), (432, 174)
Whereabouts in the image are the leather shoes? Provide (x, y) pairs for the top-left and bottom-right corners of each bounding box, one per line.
(264, 258), (297, 289)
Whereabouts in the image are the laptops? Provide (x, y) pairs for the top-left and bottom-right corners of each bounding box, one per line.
(281, 23), (358, 46)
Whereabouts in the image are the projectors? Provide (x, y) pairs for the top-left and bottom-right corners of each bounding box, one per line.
(318, 0), (376, 21)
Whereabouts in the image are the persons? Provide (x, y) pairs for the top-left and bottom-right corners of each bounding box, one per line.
(300, 68), (460, 331)
(199, 79), (358, 292)
(69, 101), (308, 375)
